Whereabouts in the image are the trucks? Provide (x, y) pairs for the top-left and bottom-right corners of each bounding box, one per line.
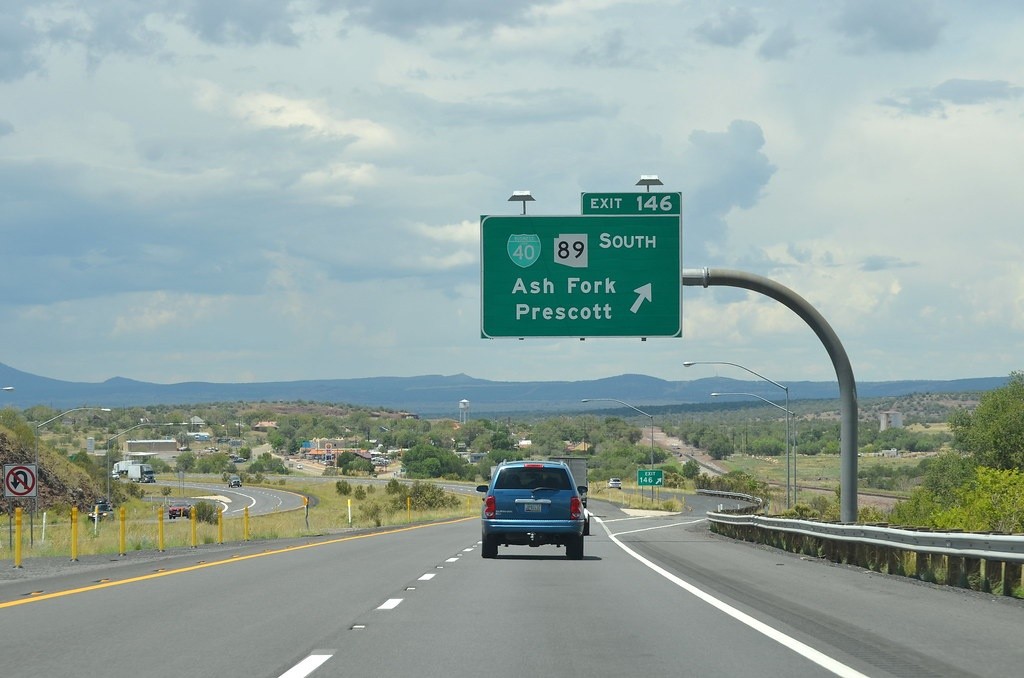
(128, 464), (155, 483)
(543, 455), (589, 536)
(114, 459), (143, 475)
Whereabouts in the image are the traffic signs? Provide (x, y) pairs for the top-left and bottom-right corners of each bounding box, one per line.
(637, 469), (664, 488)
(478, 191), (684, 340)
(637, 469), (663, 486)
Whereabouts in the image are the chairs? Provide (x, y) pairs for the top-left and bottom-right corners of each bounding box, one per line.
(544, 477), (561, 489)
(503, 474), (523, 489)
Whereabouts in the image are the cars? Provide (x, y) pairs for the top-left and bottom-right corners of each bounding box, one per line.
(607, 478), (623, 490)
(476, 459), (584, 559)
(168, 501), (191, 519)
(233, 458), (247, 463)
(112, 472), (120, 480)
(88, 498), (115, 523)
(227, 476), (242, 488)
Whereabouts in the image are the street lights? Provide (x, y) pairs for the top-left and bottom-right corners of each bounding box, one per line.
(34, 408), (111, 522)
(684, 360), (790, 514)
(581, 398), (654, 501)
(108, 422), (174, 502)
(711, 392), (796, 509)
